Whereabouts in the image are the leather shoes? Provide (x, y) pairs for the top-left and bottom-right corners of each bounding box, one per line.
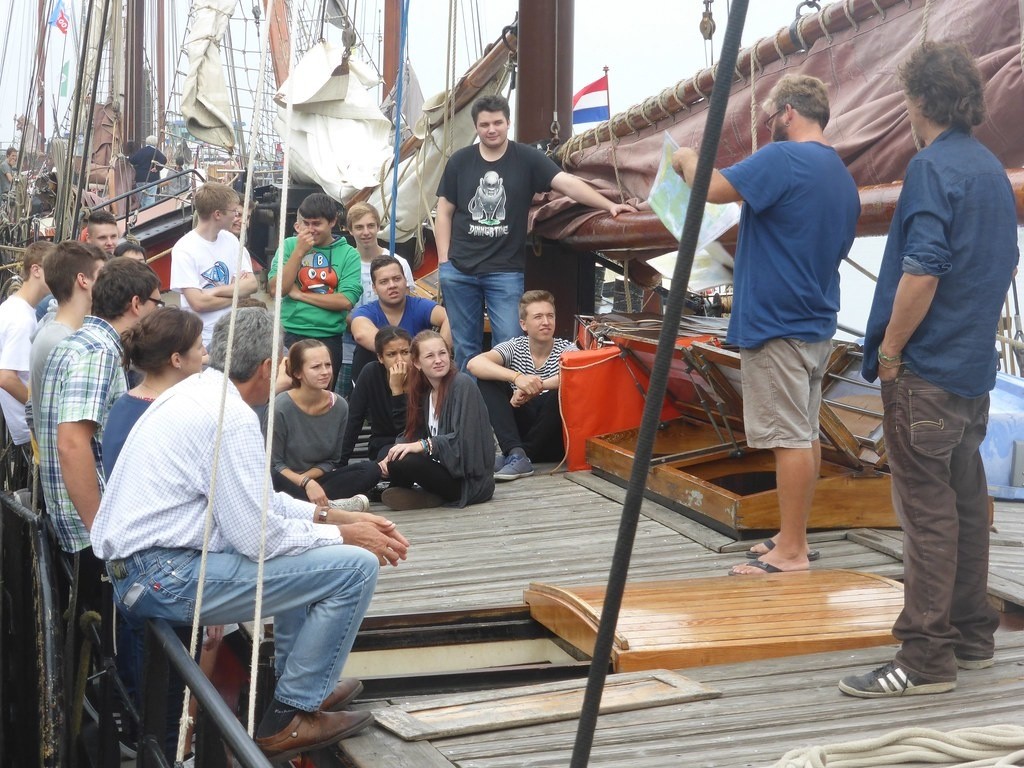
(256, 708), (374, 763)
(320, 678), (364, 711)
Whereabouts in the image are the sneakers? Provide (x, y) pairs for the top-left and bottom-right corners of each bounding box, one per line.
(328, 495), (370, 513)
(83, 686), (138, 758)
(838, 661), (956, 697)
(899, 642), (995, 670)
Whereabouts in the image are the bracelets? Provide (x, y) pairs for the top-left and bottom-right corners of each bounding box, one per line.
(300, 475), (313, 489)
(418, 437), (433, 456)
(513, 371), (522, 384)
(318, 505), (332, 524)
(878, 344), (902, 369)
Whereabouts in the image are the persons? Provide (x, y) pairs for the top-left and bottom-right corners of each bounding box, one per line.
(163, 156), (189, 196)
(0, 210), (225, 768)
(89, 306), (409, 766)
(350, 255), (454, 395)
(838, 38), (1019, 698)
(671, 72), (861, 577)
(129, 135), (167, 207)
(366, 330), (495, 511)
(0, 147), (17, 206)
(466, 290), (581, 480)
(435, 95), (638, 384)
(170, 181), (416, 512)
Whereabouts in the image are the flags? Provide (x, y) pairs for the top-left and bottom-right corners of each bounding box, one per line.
(48, 0), (70, 35)
(573, 74), (610, 124)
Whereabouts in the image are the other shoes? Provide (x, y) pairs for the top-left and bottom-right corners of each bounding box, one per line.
(380, 487), (441, 509)
(493, 455), (534, 479)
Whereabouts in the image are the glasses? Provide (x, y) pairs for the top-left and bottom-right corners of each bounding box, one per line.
(763, 107), (784, 132)
(219, 208), (238, 214)
(148, 297), (165, 310)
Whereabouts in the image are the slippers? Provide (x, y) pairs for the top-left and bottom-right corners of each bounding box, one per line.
(729, 559), (783, 576)
(745, 538), (821, 561)
